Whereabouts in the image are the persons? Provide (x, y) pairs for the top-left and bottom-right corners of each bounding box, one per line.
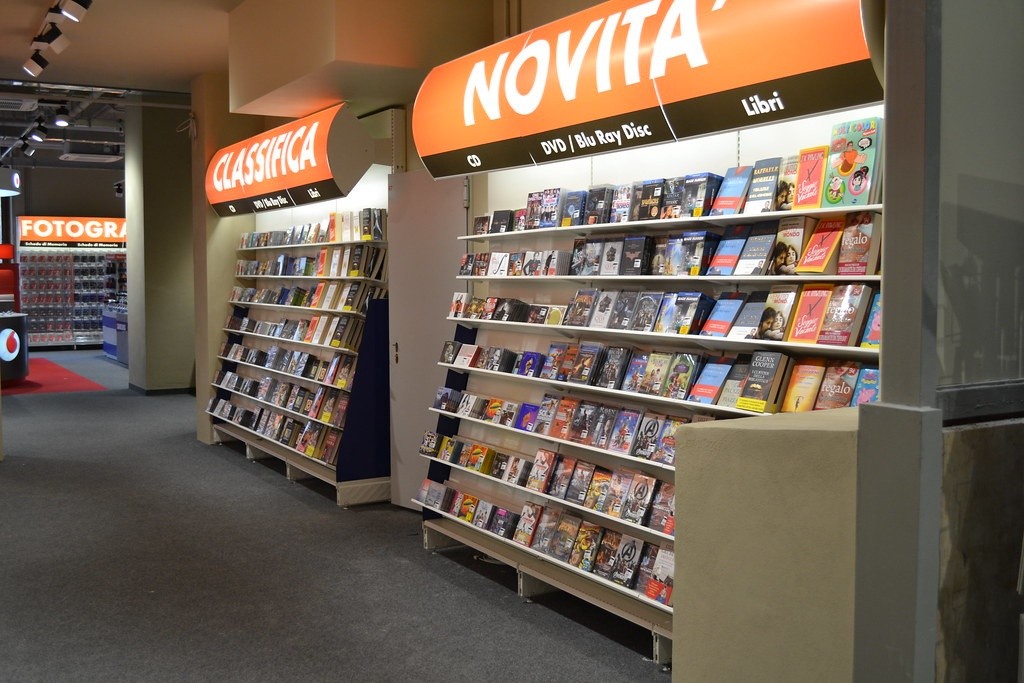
(761, 201), (770, 212)
(755, 307), (776, 339)
(540, 188), (560, 222)
(773, 181), (788, 211)
(778, 245), (798, 274)
(523, 252), (541, 275)
(545, 251), (556, 275)
(764, 311), (785, 340)
(745, 328), (756, 339)
(767, 241), (787, 275)
(751, 260), (763, 275)
(781, 183), (796, 210)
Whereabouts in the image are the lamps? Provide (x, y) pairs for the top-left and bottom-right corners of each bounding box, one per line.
(62, 0), (93, 23)
(23, 48), (48, 78)
(32, 117), (48, 142)
(20, 136), (35, 156)
(116, 183), (123, 197)
(44, 22), (70, 54)
(56, 105), (69, 127)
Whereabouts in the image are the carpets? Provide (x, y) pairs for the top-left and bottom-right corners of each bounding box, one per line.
(0, 358), (107, 395)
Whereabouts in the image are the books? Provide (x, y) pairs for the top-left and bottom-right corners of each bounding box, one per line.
(415, 117), (886, 608)
(206, 208), (387, 465)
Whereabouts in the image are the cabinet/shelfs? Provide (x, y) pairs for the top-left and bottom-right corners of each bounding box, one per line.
(411, 203), (883, 671)
(205, 240), (392, 509)
